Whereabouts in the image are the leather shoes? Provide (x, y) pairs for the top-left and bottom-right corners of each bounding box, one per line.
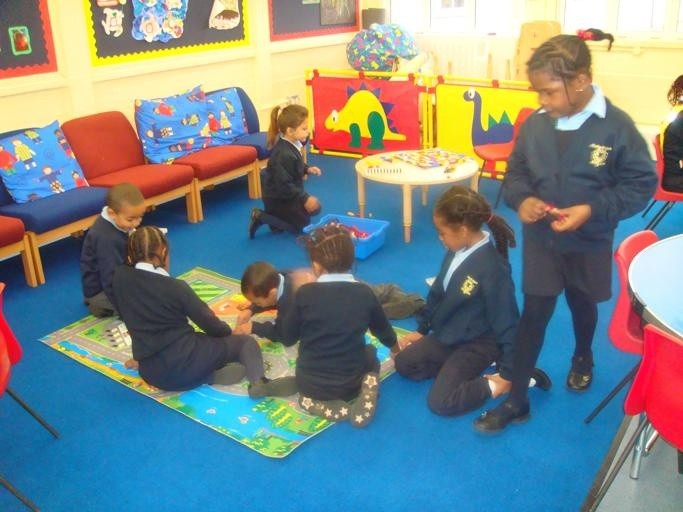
(568, 351), (594, 392)
(249, 207), (262, 238)
(474, 397), (531, 430)
(532, 368), (551, 390)
(269, 223), (283, 233)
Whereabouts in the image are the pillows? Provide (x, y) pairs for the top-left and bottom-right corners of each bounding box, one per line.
(132, 83), (236, 166)
(206, 86), (249, 137)
(0, 117), (92, 205)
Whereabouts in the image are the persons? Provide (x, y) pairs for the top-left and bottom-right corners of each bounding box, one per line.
(111, 225), (299, 400)
(247, 104), (323, 239)
(474, 27), (660, 432)
(277, 225), (401, 428)
(80, 181), (146, 316)
(662, 74), (683, 194)
(231, 260), (426, 343)
(395, 183), (552, 416)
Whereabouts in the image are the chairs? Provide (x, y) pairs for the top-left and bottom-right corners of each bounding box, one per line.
(63, 111), (198, 226)
(0, 282), (60, 512)
(204, 87), (308, 183)
(580, 322), (683, 512)
(1, 125), (114, 284)
(132, 109), (260, 221)
(641, 134), (683, 230)
(1, 215), (37, 289)
(472, 107), (536, 209)
(583, 230), (683, 474)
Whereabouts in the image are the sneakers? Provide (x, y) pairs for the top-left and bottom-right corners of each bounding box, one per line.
(248, 377), (298, 399)
(298, 396), (352, 421)
(207, 364), (248, 385)
(381, 299), (414, 319)
(351, 373), (379, 426)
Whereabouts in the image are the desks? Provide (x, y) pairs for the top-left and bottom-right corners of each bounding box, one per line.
(626, 234), (683, 478)
(354, 149), (480, 243)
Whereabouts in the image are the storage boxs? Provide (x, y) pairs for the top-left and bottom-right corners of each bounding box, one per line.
(303, 212), (390, 259)
